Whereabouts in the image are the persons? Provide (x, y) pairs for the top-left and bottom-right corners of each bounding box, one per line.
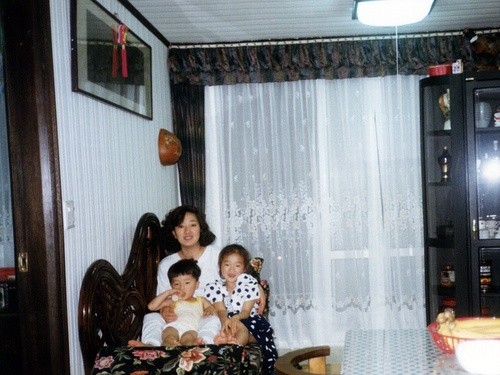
(148, 259), (215, 346)
(128, 206), (265, 348)
(204, 244), (260, 346)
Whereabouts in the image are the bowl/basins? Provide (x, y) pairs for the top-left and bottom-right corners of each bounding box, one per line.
(436, 225), (455, 239)
(426, 64), (451, 76)
(475, 102), (492, 128)
(454, 339), (500, 375)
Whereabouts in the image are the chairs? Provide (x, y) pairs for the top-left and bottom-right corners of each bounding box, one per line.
(274, 345), (330, 375)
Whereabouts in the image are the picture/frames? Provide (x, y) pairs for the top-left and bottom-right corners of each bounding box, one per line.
(71, 0), (153, 120)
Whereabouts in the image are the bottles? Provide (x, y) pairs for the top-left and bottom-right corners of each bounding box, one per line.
(440, 146), (450, 182)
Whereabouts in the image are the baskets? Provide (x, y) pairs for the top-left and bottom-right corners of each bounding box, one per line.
(428, 315), (500, 354)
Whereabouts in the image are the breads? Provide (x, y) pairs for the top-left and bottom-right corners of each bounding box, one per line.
(436, 308), (500, 351)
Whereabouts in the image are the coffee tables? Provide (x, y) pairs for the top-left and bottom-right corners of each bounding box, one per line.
(340, 328), (443, 375)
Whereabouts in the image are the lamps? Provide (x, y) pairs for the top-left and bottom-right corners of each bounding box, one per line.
(351, 0), (436, 26)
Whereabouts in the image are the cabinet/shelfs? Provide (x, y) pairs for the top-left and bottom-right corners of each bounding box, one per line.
(419, 69), (500, 326)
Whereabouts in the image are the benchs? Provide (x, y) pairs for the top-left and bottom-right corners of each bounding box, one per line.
(79, 213), (270, 375)
